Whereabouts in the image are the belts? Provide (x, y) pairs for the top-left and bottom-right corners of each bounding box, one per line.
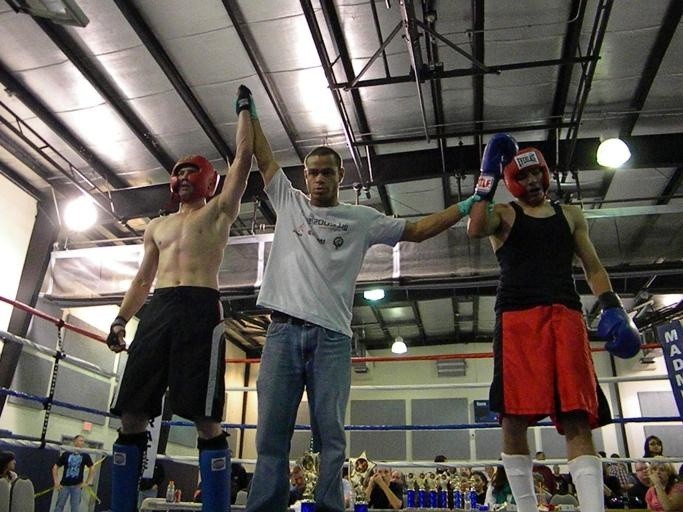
(271, 310), (317, 325)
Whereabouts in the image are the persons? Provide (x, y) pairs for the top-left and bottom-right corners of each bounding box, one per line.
(467, 134), (643, 512)
(104, 84), (256, 512)
(192, 434), (683, 511)
(0, 451), (19, 485)
(235, 83), (481, 512)
(134, 450), (165, 512)
(52, 434), (95, 512)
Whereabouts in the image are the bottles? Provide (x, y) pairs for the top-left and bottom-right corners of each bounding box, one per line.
(463, 486), (472, 511)
(165, 480), (180, 503)
(230, 476), (240, 494)
(349, 488), (356, 511)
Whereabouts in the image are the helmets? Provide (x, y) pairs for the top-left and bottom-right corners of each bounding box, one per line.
(171, 155), (220, 203)
(503, 147), (550, 207)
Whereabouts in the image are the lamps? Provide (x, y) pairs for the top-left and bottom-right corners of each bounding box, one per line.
(391, 326), (408, 354)
(595, 118), (631, 169)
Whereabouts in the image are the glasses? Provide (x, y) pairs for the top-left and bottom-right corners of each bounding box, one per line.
(649, 442), (662, 446)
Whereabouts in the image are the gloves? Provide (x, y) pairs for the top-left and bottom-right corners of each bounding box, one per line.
(474, 133), (519, 200)
(236, 84), (259, 122)
(597, 291), (641, 359)
(457, 196), (495, 215)
(106, 315), (127, 353)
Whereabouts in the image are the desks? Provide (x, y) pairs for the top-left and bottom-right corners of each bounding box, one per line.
(139, 495), (295, 512)
